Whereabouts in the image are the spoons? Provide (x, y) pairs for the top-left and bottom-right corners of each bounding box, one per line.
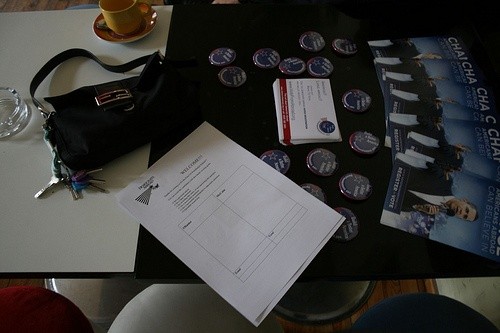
(97, 19), (107, 29)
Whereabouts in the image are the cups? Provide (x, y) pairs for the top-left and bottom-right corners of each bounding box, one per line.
(98, 0), (142, 37)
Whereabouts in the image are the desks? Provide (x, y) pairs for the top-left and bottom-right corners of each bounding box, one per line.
(137, 0), (499, 325)
(0, 5), (172, 322)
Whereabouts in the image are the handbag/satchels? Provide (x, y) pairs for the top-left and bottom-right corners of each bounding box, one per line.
(30, 47), (179, 174)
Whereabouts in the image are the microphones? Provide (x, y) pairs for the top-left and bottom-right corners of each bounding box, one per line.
(438, 209), (456, 218)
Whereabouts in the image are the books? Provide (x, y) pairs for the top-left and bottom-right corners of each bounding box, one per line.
(369, 35), (500, 261)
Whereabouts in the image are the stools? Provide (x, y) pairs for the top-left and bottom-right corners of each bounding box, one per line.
(345, 293), (500, 333)
(0, 287), (94, 333)
(106, 283), (285, 333)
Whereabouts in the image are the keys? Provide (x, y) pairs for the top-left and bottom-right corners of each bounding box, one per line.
(34, 168), (107, 201)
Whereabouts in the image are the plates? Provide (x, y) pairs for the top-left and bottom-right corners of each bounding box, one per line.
(92, 2), (158, 44)
